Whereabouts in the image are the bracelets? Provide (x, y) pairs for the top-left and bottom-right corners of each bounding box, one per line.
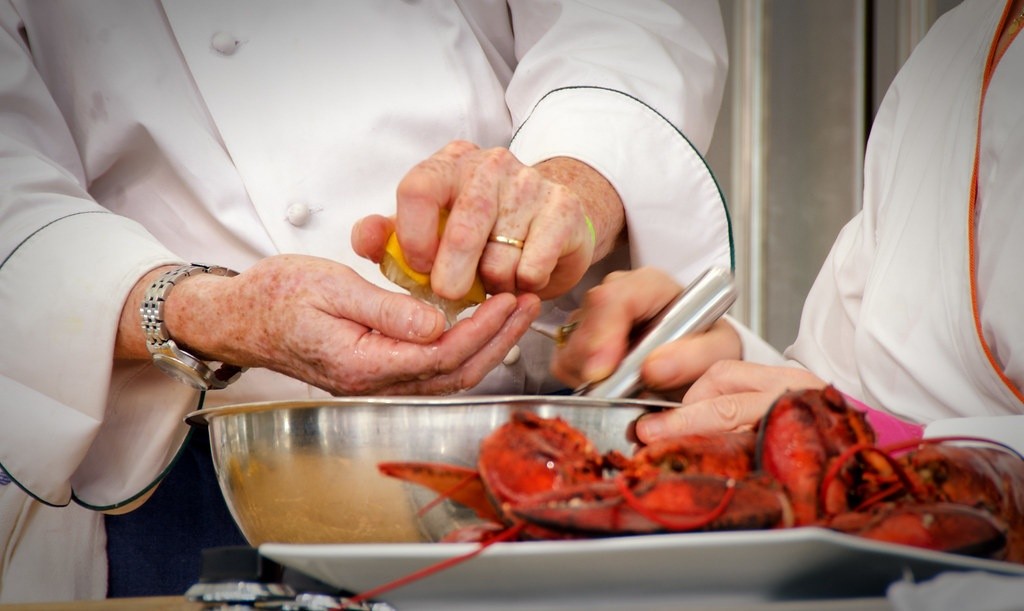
(142, 262), (252, 392)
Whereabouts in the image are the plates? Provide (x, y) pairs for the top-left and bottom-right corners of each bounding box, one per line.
(259, 526), (1024, 611)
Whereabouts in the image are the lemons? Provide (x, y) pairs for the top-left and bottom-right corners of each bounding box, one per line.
(378, 211), (487, 323)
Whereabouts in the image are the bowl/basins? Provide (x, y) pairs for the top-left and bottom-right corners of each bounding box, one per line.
(184, 395), (684, 550)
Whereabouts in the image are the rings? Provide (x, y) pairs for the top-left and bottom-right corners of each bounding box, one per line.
(488, 233), (528, 250)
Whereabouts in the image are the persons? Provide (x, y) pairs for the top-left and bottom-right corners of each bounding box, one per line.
(1, 0), (1022, 598)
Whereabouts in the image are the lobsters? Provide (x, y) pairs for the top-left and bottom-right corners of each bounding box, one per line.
(329, 385), (1024, 611)
